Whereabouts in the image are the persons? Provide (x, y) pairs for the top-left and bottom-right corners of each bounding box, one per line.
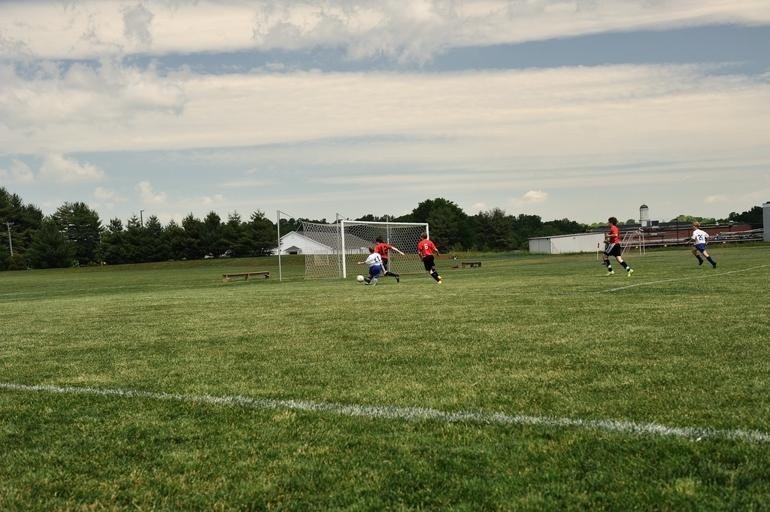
(417, 232), (443, 284)
(603, 215), (634, 278)
(686, 220), (717, 269)
(357, 246), (383, 285)
(364, 235), (406, 283)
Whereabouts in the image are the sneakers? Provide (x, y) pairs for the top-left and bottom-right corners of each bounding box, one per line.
(712, 261), (718, 269)
(699, 259), (704, 265)
(437, 275), (443, 284)
(606, 270), (615, 276)
(627, 268), (633, 278)
(364, 278), (370, 283)
(395, 273), (399, 282)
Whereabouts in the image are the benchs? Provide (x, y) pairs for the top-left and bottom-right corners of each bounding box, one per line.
(223, 271), (269, 282)
(457, 262), (481, 268)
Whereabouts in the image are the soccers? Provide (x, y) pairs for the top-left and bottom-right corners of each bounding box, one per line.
(357, 274), (365, 282)
